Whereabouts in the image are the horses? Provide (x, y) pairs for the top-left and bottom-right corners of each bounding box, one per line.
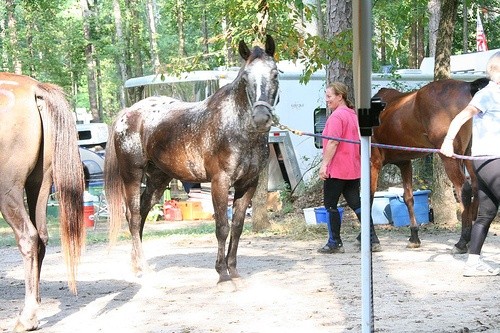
(102, 33), (282, 292)
(356, 78), (490, 255)
(0, 71), (86, 333)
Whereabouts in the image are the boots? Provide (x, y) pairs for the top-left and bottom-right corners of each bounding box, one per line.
(355, 207), (382, 252)
(316, 210), (345, 255)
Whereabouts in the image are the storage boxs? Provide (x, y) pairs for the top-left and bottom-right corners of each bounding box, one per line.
(176, 201), (201, 220)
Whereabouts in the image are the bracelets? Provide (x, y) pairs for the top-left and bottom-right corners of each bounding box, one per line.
(444, 136), (454, 142)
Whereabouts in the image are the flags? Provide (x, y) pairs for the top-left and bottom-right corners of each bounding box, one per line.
(477, 9), (488, 51)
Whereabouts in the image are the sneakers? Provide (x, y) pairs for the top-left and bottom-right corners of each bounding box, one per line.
(462, 261), (497, 276)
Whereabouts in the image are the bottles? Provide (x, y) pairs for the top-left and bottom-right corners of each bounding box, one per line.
(165, 197), (183, 221)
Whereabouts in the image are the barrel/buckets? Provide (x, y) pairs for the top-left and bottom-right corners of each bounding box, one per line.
(370, 192), (391, 225)
(383, 190), (431, 228)
(83, 201), (95, 229)
(301, 208), (342, 226)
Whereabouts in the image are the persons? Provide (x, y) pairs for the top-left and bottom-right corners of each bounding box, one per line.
(440, 51), (500, 277)
(317, 82), (382, 254)
